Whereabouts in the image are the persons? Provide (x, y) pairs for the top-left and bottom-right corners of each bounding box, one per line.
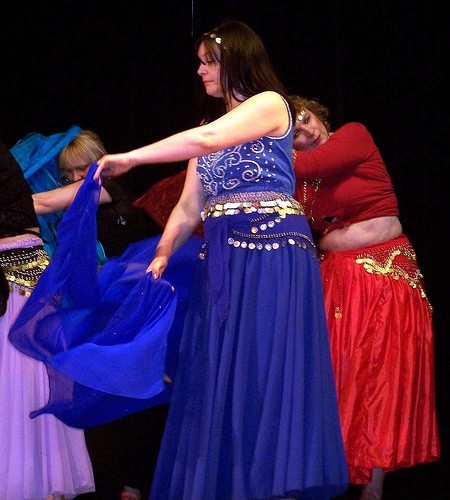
(285, 93), (442, 500)
(94, 16), (349, 499)
(0, 139), (96, 500)
(32, 131), (162, 500)
